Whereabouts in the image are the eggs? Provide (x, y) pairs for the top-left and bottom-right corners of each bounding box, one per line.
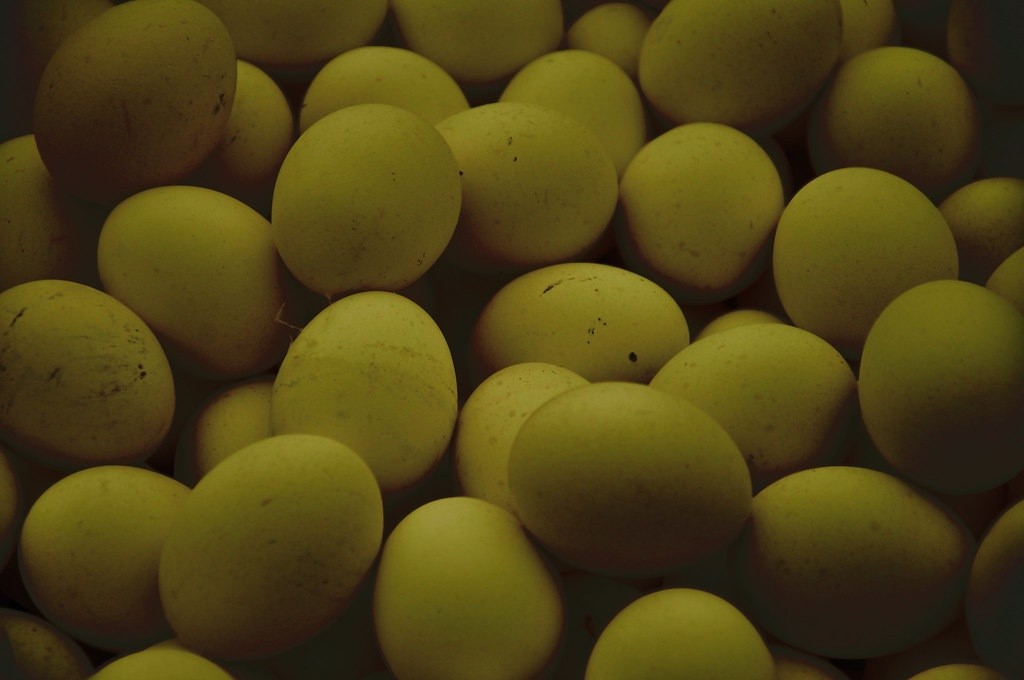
(0, 0), (1024, 680)
